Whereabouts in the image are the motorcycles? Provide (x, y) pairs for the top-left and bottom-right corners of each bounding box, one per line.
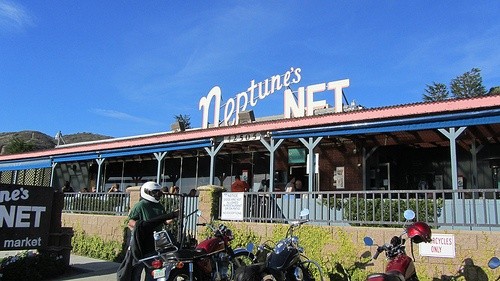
(138, 208), (323, 281)
(364, 209), (420, 281)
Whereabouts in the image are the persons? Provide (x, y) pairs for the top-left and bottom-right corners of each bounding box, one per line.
(256, 178), (275, 192)
(284, 177), (297, 195)
(89, 186), (101, 198)
(76, 188), (91, 198)
(230, 176), (248, 192)
(107, 184), (125, 198)
(124, 184), (133, 196)
(61, 181), (74, 197)
(117, 181), (179, 281)
(168, 184), (180, 200)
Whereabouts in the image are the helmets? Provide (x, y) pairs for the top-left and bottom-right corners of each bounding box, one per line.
(407, 221), (432, 243)
(140, 181), (162, 202)
